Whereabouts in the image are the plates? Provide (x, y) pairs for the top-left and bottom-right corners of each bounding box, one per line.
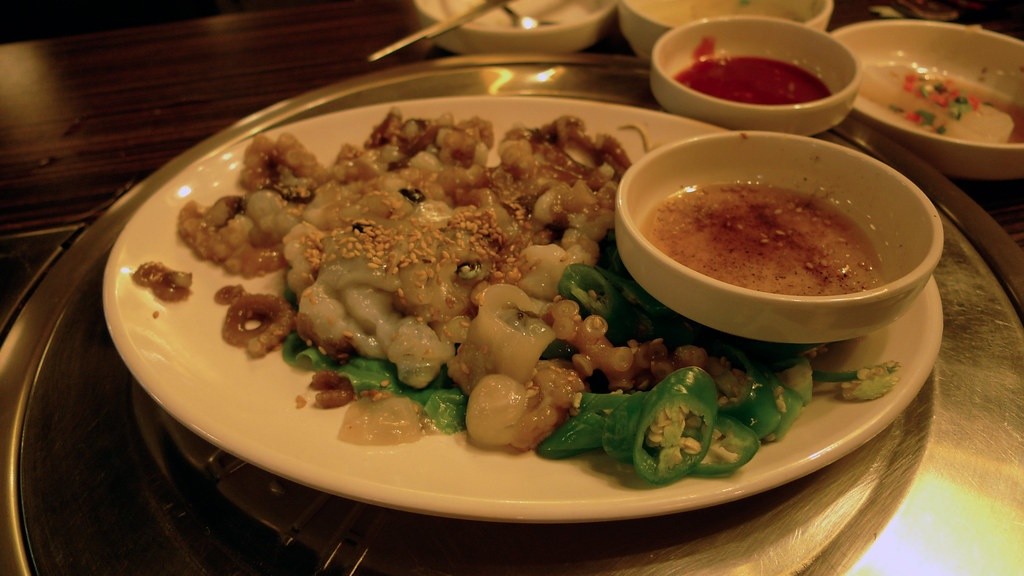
(103, 94), (945, 526)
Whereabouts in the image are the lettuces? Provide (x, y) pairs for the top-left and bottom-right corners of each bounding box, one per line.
(280, 271), (473, 433)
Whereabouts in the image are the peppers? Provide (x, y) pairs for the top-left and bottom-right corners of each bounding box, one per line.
(533, 262), (897, 484)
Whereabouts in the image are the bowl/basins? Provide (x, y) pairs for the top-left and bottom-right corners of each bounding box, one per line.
(830, 18), (1024, 180)
(647, 13), (863, 136)
(614, 131), (945, 345)
(618, 0), (834, 59)
(412, 0), (617, 55)
